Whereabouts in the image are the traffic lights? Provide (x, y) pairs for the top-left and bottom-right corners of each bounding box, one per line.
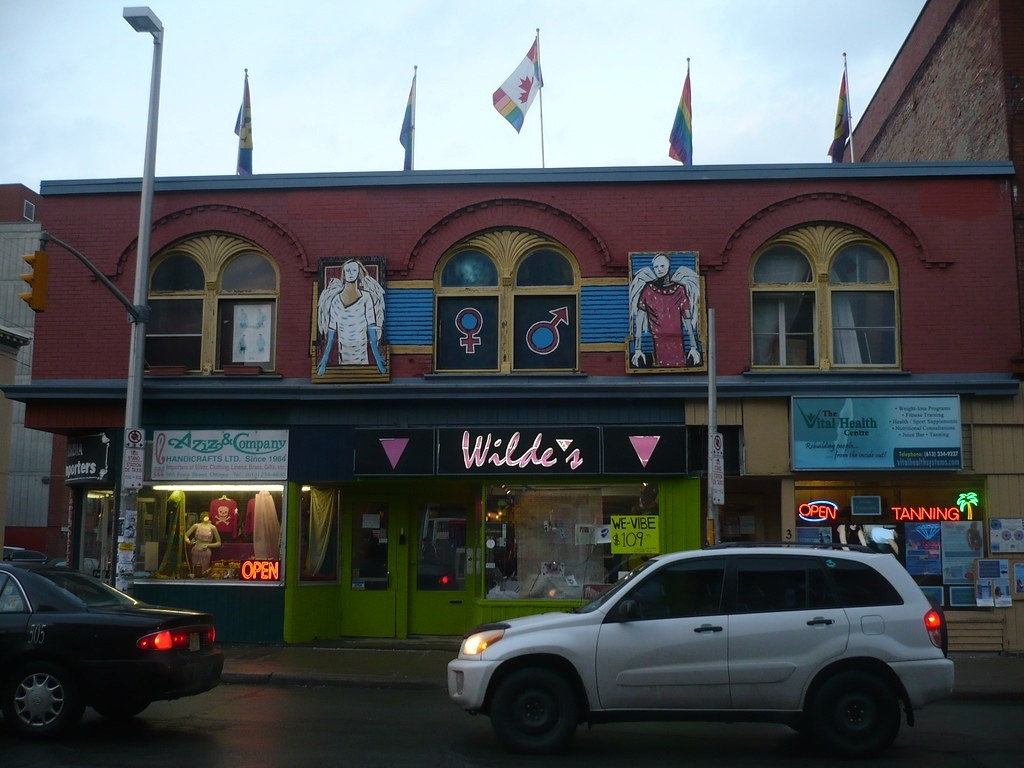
(18, 251), (50, 314)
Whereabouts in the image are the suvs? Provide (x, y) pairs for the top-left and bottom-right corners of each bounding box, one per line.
(447, 540), (956, 755)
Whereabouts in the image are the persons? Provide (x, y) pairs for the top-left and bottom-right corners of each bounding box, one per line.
(836, 525), (898, 554)
(185, 517), (222, 571)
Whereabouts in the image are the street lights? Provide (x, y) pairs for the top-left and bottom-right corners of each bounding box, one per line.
(104, 4), (168, 590)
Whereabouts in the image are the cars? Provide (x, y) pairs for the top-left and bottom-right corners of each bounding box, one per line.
(0, 561), (226, 744)
(2, 546), (48, 569)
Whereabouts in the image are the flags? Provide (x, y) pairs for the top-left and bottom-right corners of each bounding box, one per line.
(493, 38), (544, 135)
(234, 80), (253, 174)
(399, 85), (412, 170)
(669, 74), (693, 165)
(828, 70), (849, 163)
